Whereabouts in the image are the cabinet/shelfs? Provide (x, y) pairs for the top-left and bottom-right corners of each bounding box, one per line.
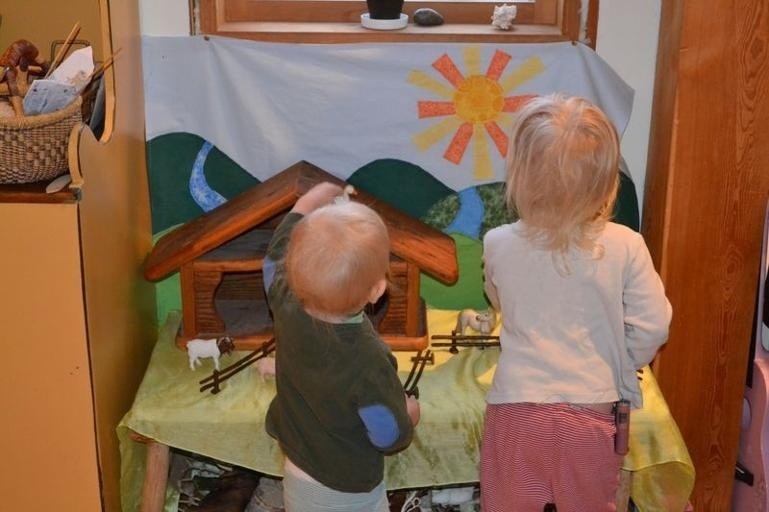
(1, 0), (157, 511)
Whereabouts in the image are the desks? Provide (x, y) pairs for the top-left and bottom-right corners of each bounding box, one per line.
(116, 308), (698, 512)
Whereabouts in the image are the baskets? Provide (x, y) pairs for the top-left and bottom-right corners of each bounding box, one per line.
(1, 42), (101, 184)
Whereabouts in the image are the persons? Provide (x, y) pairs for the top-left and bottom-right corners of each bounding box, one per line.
(481, 91), (674, 512)
(263, 181), (421, 512)
